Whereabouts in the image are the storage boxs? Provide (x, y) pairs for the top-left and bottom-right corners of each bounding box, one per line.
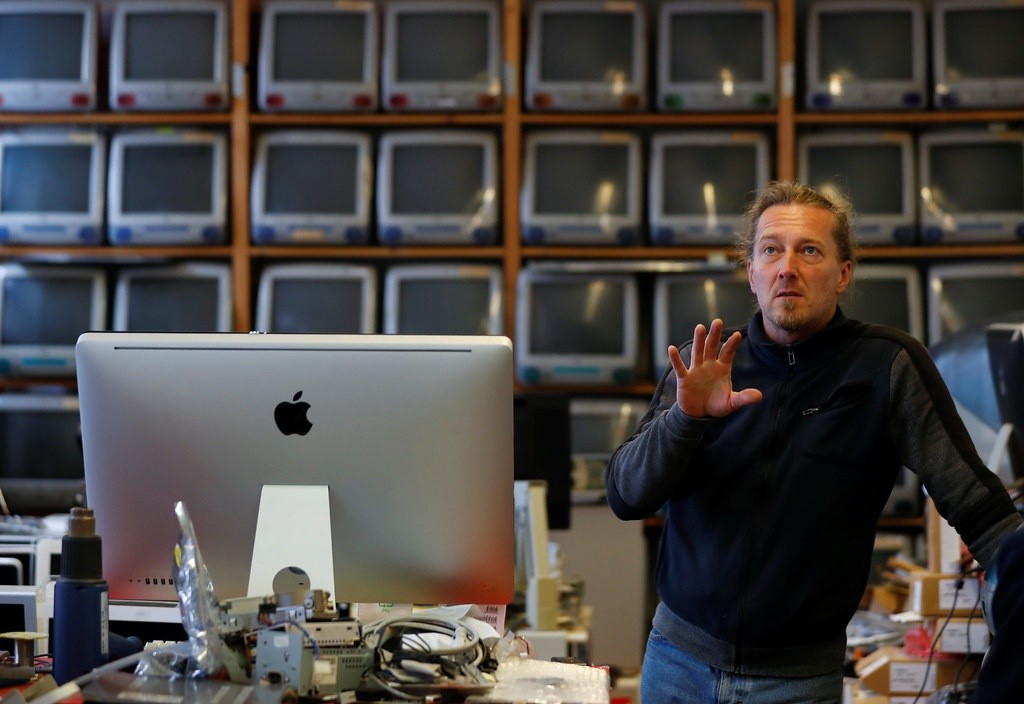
(840, 496), (992, 704)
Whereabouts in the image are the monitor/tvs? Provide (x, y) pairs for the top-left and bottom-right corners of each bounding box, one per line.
(0, 2), (1024, 624)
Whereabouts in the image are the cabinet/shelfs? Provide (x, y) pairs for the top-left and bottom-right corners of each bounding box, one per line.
(0, 0), (1024, 401)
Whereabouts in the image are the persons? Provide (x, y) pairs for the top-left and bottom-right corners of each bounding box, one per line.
(604, 183), (1024, 704)
